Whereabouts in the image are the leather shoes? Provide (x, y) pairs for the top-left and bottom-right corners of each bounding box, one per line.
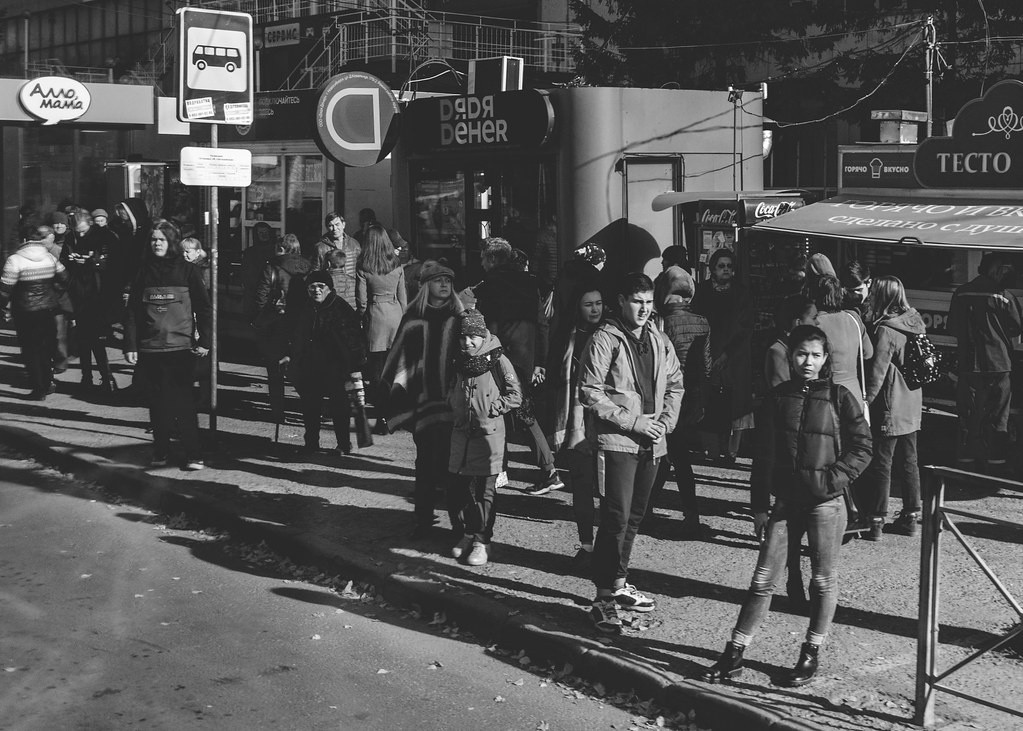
(789, 643), (819, 686)
(701, 642), (743, 683)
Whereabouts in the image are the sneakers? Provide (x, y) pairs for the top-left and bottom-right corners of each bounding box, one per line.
(524, 468), (565, 495)
(869, 521), (882, 541)
(452, 533), (492, 566)
(591, 595), (624, 633)
(611, 583), (655, 613)
(893, 509), (918, 537)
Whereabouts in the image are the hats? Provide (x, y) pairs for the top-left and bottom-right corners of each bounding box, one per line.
(51, 212), (69, 228)
(456, 308), (487, 338)
(420, 266), (455, 285)
(92, 209), (108, 219)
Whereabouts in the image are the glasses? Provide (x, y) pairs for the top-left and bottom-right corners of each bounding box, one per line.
(308, 284), (325, 289)
(716, 263), (732, 268)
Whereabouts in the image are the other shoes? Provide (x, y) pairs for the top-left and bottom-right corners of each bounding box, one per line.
(80, 377), (93, 389)
(568, 544), (594, 570)
(185, 447), (204, 471)
(373, 418), (389, 434)
(24, 385), (55, 401)
(99, 380), (119, 396)
(151, 451), (168, 466)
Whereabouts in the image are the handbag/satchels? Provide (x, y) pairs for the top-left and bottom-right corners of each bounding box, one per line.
(863, 400), (871, 428)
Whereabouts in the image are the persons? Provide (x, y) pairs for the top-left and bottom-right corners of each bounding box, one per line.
(946, 253), (1023, 464)
(387, 237), (926, 574)
(0, 198), (423, 469)
(578, 272), (685, 632)
(699, 325), (872, 686)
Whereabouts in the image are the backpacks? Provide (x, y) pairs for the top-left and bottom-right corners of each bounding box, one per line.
(881, 325), (943, 390)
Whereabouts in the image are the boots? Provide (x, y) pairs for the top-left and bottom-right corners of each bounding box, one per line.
(958, 439), (974, 462)
(987, 431), (1007, 465)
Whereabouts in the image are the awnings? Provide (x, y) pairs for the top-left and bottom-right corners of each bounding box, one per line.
(753, 193), (1023, 252)
